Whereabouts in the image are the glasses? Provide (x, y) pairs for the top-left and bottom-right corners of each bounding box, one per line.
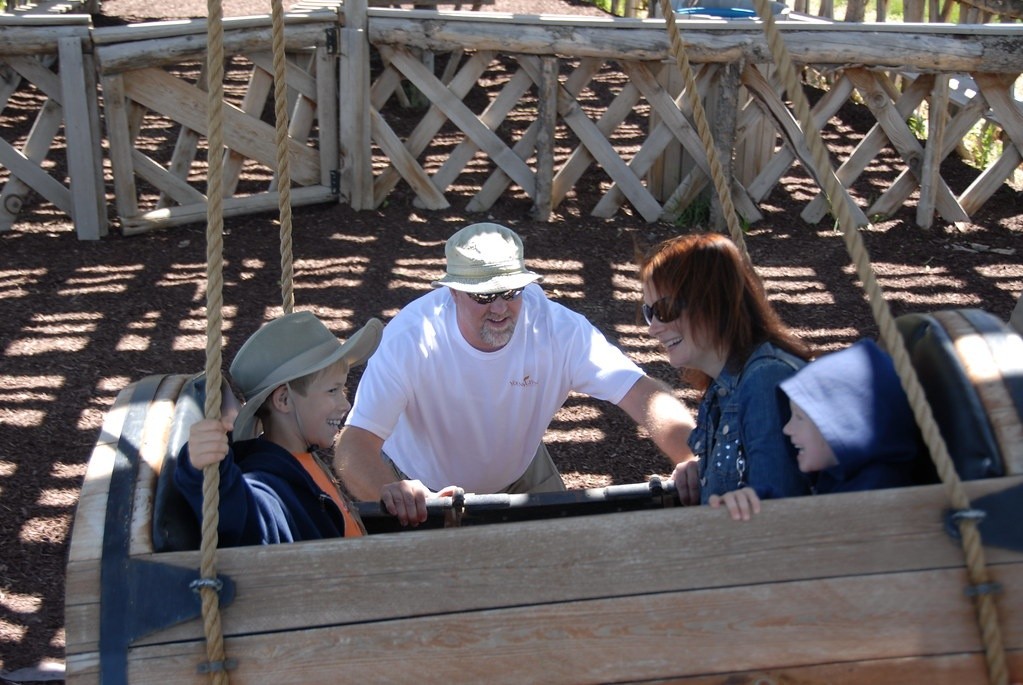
(642, 295), (686, 327)
(466, 287), (524, 304)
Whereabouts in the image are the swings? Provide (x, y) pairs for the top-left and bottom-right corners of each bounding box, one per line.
(64, 0), (1023, 685)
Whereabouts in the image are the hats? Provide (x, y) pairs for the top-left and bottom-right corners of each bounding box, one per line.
(430, 222), (544, 294)
(229, 309), (383, 452)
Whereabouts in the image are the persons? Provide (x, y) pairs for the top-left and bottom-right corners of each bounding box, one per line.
(775, 338), (910, 494)
(333, 223), (700, 527)
(638, 235), (811, 521)
(177, 311), (368, 545)
